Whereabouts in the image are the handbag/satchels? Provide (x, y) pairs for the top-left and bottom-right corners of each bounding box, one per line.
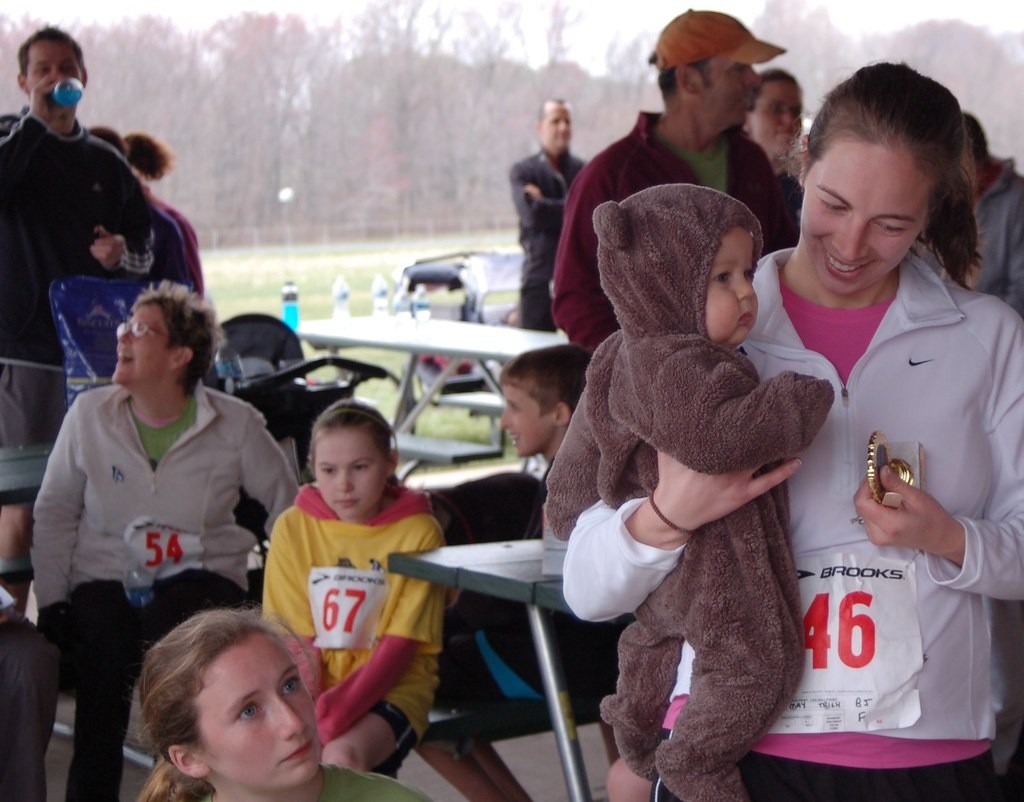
(47, 273), (196, 416)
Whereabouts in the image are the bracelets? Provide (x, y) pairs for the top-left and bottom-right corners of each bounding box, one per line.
(650, 490), (693, 534)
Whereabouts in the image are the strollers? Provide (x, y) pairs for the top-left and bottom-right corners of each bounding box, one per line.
(204, 308), (395, 487)
(389, 262), (496, 403)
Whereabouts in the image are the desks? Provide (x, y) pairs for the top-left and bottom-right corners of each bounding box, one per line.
(299, 316), (570, 449)
(385, 540), (632, 801)
(0, 441), (55, 506)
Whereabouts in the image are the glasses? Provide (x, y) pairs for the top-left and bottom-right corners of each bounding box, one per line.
(117, 321), (176, 340)
(750, 101), (803, 117)
(26, 63), (78, 76)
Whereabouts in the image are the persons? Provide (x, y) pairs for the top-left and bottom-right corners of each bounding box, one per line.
(0, 28), (202, 606)
(742, 69), (804, 161)
(410, 344), (634, 802)
(512, 97), (586, 328)
(553, 10), (800, 344)
(133, 607), (420, 802)
(258, 400), (441, 770)
(547, 181), (836, 802)
(539, 61), (1024, 802)
(953, 107), (1024, 307)
(0, 280), (299, 801)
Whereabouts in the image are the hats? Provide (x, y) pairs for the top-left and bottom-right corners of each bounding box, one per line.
(645, 7), (788, 72)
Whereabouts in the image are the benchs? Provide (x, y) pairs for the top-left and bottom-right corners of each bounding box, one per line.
(386, 390), (507, 465)
(429, 696), (603, 742)
(0, 554), (33, 582)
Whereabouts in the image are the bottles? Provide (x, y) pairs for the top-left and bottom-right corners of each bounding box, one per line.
(281, 281), (300, 331)
(122, 556), (158, 629)
(215, 339), (246, 390)
(47, 76), (84, 107)
(332, 276), (350, 326)
(394, 278), (431, 322)
(372, 272), (389, 321)
